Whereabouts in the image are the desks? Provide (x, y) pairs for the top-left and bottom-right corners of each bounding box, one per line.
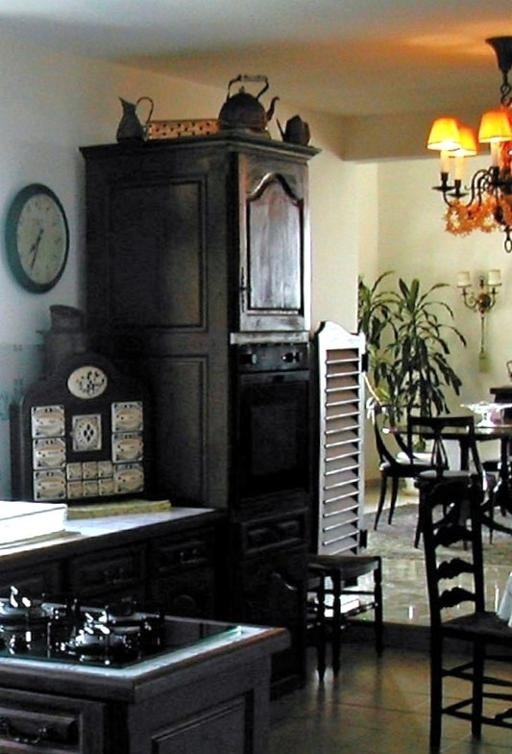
(0, 598), (293, 754)
(0, 499), (220, 626)
(381, 417), (511, 548)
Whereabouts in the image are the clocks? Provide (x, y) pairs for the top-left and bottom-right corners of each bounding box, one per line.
(4, 183), (72, 297)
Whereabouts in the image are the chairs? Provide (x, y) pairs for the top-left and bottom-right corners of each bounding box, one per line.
(409, 469), (511, 754)
(370, 401), (447, 530)
(278, 552), (385, 676)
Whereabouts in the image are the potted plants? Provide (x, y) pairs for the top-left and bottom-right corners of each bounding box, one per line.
(352, 269), (465, 495)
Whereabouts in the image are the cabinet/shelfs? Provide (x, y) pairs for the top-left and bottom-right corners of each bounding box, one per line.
(75, 131), (316, 703)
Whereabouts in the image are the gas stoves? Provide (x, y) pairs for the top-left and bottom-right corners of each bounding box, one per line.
(0, 584), (241, 671)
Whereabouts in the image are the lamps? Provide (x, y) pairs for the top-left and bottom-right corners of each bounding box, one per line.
(423, 31), (511, 249)
(454, 267), (501, 371)
